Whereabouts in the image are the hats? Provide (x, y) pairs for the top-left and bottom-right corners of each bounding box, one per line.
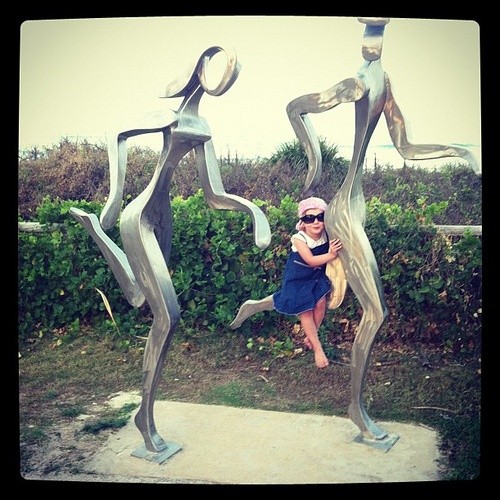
(295, 196), (327, 231)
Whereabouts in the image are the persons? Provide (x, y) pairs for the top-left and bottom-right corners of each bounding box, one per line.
(272, 197), (343, 368)
(228, 18), (480, 441)
(65, 40), (271, 451)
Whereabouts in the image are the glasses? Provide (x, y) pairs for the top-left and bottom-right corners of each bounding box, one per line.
(299, 211), (325, 224)
